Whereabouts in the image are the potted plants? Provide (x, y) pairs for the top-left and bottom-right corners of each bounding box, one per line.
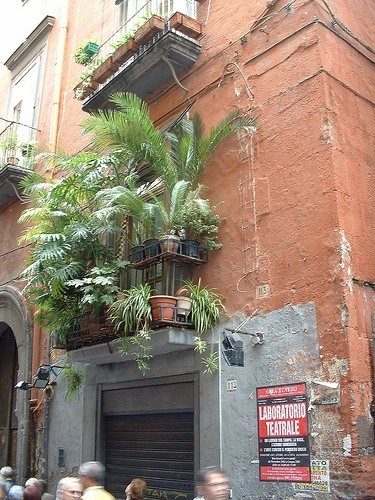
(86, 51), (119, 83)
(72, 71), (97, 101)
(14, 92), (261, 407)
(0, 128), (23, 165)
(130, 8), (165, 45)
(72, 40), (99, 64)
(109, 32), (138, 65)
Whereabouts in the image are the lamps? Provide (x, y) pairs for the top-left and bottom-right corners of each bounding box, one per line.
(32, 361), (65, 388)
(14, 381), (56, 391)
(220, 328), (264, 368)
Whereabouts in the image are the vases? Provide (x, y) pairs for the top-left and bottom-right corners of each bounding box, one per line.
(168, 10), (203, 39)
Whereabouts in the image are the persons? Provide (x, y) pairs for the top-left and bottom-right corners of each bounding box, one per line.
(193, 465), (231, 500)
(56, 476), (84, 500)
(77, 461), (116, 500)
(0, 466), (55, 500)
(125, 478), (147, 500)
